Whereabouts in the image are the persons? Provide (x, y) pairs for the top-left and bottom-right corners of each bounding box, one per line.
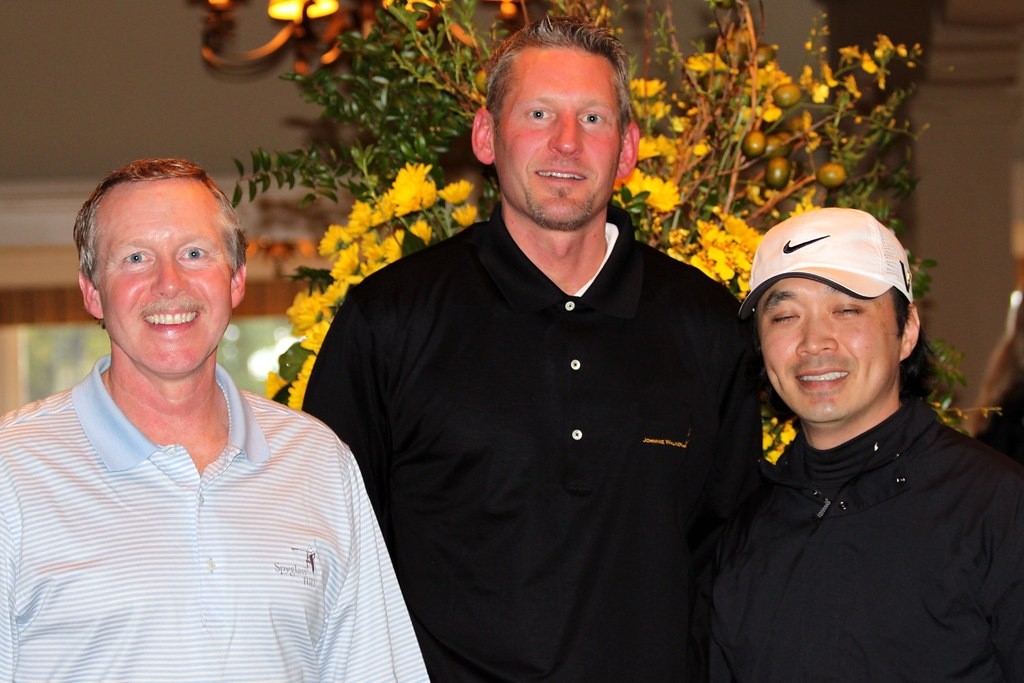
(964, 286), (1024, 465)
(685, 205), (1023, 683)
(299, 11), (768, 683)
(0, 154), (434, 683)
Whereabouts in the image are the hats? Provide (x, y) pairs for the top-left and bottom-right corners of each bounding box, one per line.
(736, 205), (914, 319)
